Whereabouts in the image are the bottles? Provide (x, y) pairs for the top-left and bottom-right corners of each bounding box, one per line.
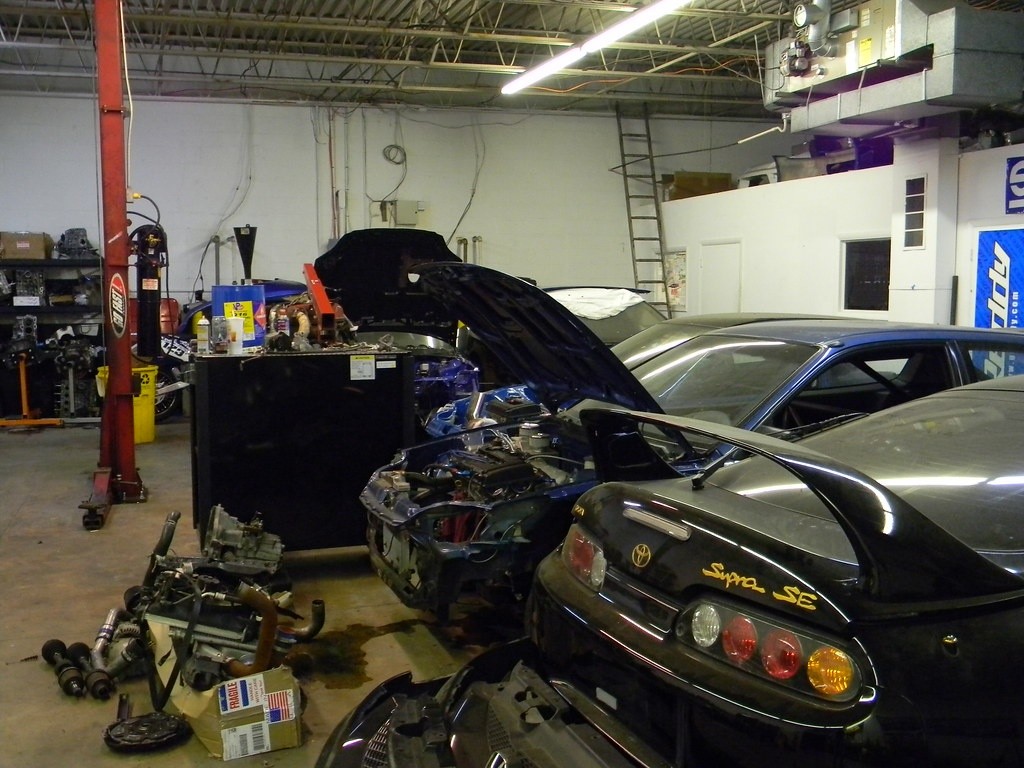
(276, 308), (290, 337)
(229, 317), (245, 354)
(195, 315), (210, 353)
(211, 315), (226, 350)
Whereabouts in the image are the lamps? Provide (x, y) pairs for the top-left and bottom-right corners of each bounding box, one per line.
(495, 2), (690, 94)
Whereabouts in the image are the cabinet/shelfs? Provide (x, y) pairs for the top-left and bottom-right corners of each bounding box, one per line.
(0, 257), (107, 414)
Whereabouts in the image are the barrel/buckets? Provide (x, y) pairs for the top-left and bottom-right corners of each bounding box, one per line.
(96, 364), (158, 446)
(210, 284), (267, 348)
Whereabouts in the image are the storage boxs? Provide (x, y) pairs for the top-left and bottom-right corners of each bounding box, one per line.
(1, 231), (55, 261)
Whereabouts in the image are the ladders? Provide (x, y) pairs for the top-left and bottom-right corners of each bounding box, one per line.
(614, 99), (672, 322)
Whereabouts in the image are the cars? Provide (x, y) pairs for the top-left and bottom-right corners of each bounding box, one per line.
(314, 224), (1022, 641)
(520, 363), (1024, 767)
